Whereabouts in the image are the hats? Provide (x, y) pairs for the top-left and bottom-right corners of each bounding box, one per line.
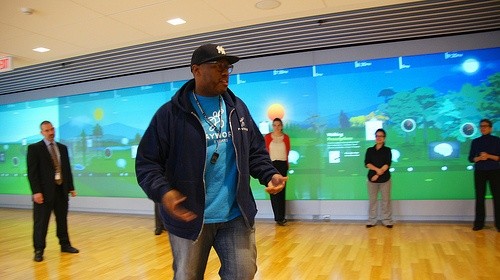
(190, 44), (238, 71)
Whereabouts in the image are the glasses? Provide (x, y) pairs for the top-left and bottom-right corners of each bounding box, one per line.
(206, 61), (233, 74)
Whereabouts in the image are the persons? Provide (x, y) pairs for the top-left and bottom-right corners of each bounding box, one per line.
(136, 44), (287, 280)
(364, 129), (392, 229)
(468, 119), (500, 232)
(263, 118), (291, 227)
(27, 121), (78, 262)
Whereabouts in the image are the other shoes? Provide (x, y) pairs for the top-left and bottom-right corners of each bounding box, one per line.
(473, 226), (482, 231)
(277, 219), (286, 225)
(155, 226), (163, 235)
(61, 247), (78, 254)
(34, 250), (43, 262)
(387, 224), (393, 228)
(366, 225), (372, 228)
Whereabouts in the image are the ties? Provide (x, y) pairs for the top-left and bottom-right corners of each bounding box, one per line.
(49, 142), (62, 185)
